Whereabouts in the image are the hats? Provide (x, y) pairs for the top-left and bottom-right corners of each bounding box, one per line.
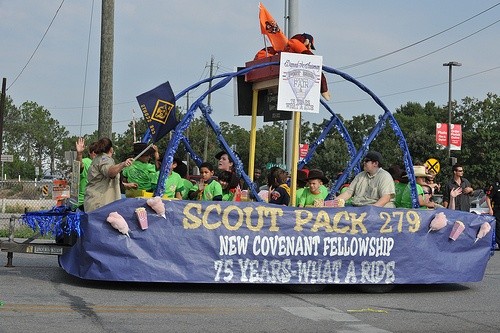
(157, 152), (182, 163)
(402, 165), (432, 177)
(366, 150), (383, 167)
(215, 150), (241, 160)
(304, 169), (329, 185)
(132, 143), (156, 156)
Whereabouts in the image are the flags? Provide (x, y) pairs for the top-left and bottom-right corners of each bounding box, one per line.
(136, 81), (178, 144)
(259, 2), (288, 51)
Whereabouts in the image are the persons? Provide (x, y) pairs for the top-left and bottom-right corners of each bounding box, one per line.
(333, 151), (395, 208)
(395, 166), (462, 210)
(476, 183), (500, 249)
(291, 33), (315, 50)
(120, 172), (138, 198)
(151, 151), (184, 199)
(188, 162), (223, 201)
(121, 143), (159, 192)
(254, 46), (277, 59)
(215, 150), (241, 201)
(84, 138), (138, 212)
(243, 166), (291, 206)
(443, 165), (474, 212)
(76, 138), (96, 211)
(296, 167), (330, 208)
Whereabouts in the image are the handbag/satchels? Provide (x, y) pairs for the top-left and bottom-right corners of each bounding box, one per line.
(55, 207), (78, 246)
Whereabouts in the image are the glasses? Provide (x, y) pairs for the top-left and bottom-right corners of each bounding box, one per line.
(458, 170), (464, 172)
(362, 158), (372, 162)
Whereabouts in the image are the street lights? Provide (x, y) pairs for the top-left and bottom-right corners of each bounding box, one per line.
(443, 61), (462, 203)
